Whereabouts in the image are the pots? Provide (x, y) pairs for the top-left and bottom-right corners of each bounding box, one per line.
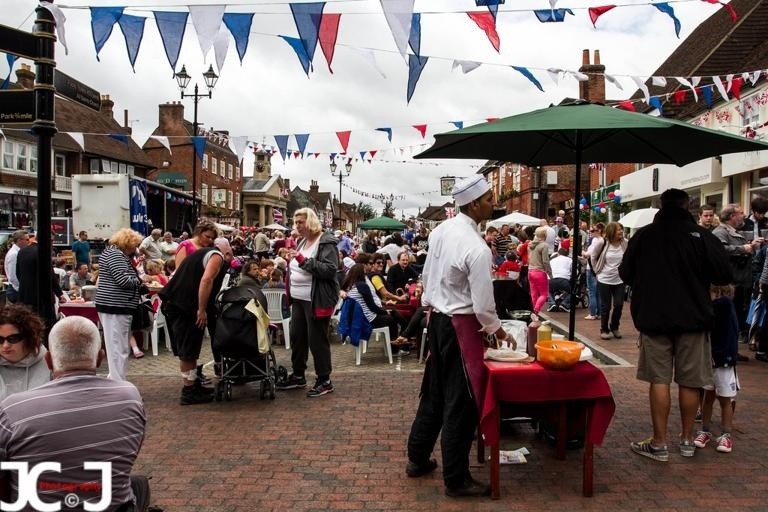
(506, 309), (531, 320)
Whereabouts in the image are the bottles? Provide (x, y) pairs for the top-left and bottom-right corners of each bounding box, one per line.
(528, 315), (552, 359)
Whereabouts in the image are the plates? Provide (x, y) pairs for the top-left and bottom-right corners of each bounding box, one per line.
(487, 349), (529, 362)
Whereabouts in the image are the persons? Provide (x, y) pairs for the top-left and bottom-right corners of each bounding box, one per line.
(0, 172), (766, 512)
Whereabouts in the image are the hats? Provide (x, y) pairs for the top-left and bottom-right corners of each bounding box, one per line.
(451, 174), (490, 206)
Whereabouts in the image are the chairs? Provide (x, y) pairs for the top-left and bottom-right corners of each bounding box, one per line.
(1, 230), (533, 365)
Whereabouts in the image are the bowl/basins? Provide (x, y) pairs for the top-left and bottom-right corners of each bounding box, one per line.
(534, 340), (584, 370)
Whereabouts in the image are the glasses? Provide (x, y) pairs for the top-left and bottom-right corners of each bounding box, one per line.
(0, 334), (22, 344)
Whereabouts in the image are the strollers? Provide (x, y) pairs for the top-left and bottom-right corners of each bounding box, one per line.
(213, 285), (289, 401)
(554, 257), (590, 310)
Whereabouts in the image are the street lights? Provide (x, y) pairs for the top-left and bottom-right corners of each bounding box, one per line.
(173, 62), (219, 234)
(328, 157), (352, 232)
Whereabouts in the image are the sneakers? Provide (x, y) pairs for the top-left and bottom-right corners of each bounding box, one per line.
(391, 337), (409, 345)
(276, 374), (306, 389)
(601, 329), (622, 339)
(585, 314), (601, 319)
(406, 458), (437, 475)
(306, 378), (334, 397)
(547, 305), (556, 311)
(181, 364), (215, 405)
(695, 409), (703, 423)
(446, 480), (491, 497)
(632, 432), (733, 461)
(393, 350), (410, 357)
(559, 304), (570, 313)
(132, 347), (144, 358)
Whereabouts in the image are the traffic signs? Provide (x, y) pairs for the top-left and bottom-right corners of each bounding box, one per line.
(53, 67), (105, 111)
(0, 88), (36, 123)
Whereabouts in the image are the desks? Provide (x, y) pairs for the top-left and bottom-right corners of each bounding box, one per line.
(475, 330), (617, 497)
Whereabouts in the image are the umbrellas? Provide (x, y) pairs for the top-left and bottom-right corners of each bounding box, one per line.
(411, 98), (766, 341)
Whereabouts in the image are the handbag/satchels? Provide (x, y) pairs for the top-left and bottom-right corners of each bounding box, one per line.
(131, 300), (155, 330)
